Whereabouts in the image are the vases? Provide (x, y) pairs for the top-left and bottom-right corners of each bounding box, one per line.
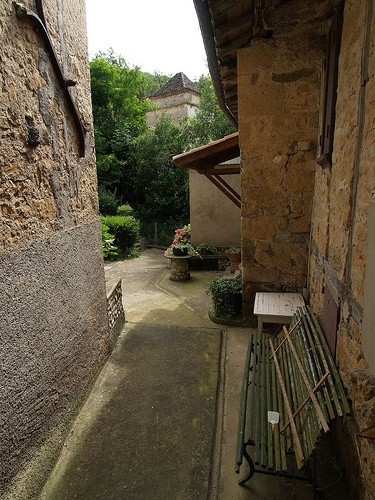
(173, 247), (188, 256)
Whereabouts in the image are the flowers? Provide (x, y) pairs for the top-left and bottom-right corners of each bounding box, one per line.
(172, 227), (202, 259)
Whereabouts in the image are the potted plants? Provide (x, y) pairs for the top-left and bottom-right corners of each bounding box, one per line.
(226, 245), (242, 273)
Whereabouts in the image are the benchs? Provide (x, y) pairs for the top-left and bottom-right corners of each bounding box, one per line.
(233, 304), (354, 499)
